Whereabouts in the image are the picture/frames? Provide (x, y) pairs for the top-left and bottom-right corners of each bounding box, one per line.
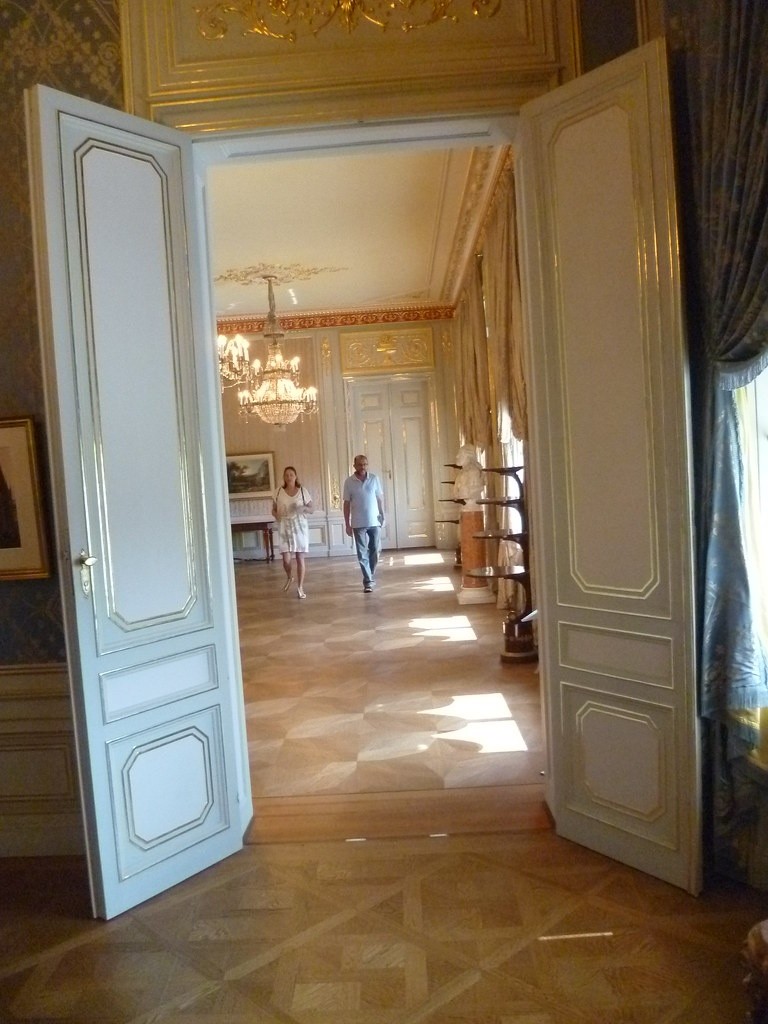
(0, 419), (51, 580)
(226, 451), (276, 502)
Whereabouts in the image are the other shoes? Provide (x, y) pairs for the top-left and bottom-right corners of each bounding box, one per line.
(297, 587), (306, 598)
(365, 584), (372, 592)
(284, 577), (293, 590)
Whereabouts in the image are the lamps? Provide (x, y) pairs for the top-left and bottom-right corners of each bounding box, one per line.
(237, 275), (318, 430)
(214, 332), (262, 393)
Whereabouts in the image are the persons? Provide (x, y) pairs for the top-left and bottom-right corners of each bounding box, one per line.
(272, 467), (315, 599)
(344, 455), (386, 592)
(453, 443), (485, 512)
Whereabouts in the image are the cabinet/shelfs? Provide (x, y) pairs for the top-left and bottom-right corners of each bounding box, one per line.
(466, 467), (539, 665)
(434, 463), (466, 571)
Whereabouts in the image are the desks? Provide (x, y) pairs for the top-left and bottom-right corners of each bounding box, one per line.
(231, 521), (275, 564)
